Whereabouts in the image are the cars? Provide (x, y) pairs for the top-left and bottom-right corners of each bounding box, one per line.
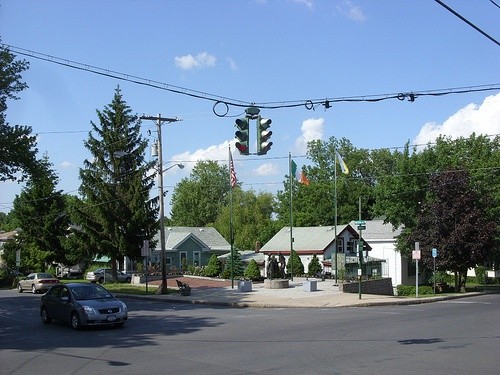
(86, 269), (131, 283)
(41, 283), (128, 330)
(17, 272), (59, 294)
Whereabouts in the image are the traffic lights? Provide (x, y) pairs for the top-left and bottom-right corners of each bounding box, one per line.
(257, 119), (272, 155)
(235, 118), (249, 155)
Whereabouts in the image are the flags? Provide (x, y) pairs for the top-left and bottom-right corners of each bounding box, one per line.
(289, 157), (309, 185)
(228, 150), (237, 186)
(335, 150), (349, 175)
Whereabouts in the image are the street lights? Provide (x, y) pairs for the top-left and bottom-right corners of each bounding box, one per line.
(157, 164), (184, 287)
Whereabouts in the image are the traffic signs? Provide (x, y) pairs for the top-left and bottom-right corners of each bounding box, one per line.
(354, 220), (366, 225)
(358, 226), (366, 229)
